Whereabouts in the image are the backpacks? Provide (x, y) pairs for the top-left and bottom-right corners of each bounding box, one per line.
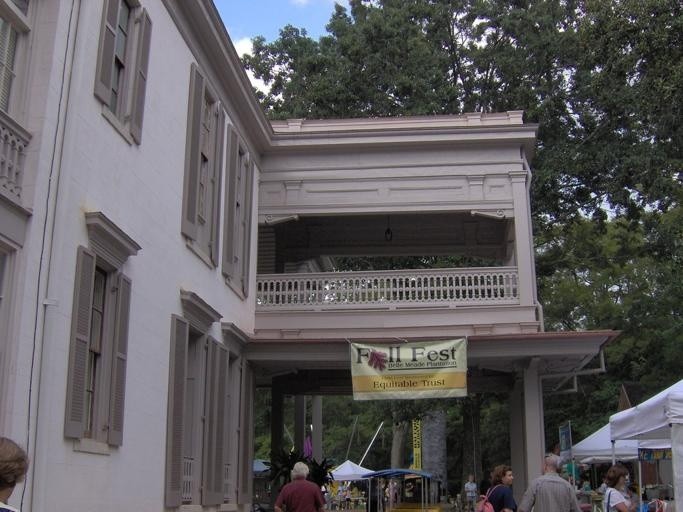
(475, 495), (494, 512)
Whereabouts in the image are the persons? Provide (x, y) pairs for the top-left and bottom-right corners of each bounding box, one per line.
(464, 454), (584, 512)
(274, 462), (326, 512)
(337, 486), (353, 510)
(604, 463), (638, 512)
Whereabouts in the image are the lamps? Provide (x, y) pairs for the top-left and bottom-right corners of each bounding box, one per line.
(385, 214), (394, 243)
